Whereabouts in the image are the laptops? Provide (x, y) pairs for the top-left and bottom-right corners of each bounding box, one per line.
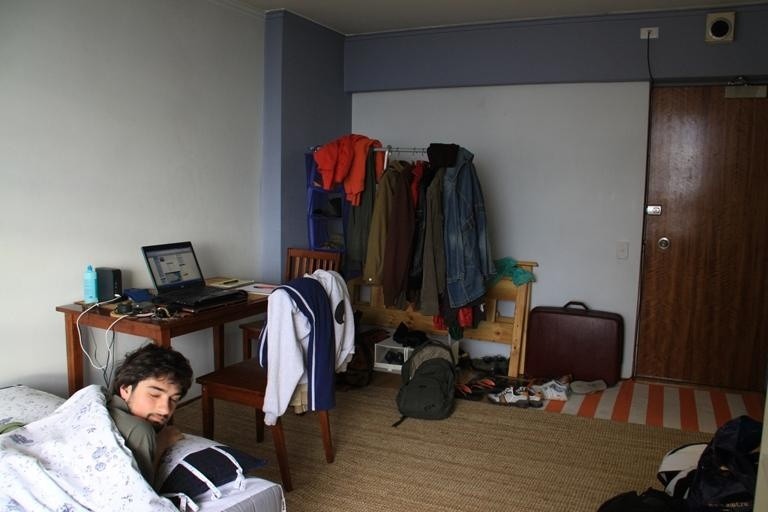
(141, 241), (248, 310)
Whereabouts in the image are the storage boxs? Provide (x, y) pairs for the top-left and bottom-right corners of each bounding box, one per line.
(373, 334), (459, 376)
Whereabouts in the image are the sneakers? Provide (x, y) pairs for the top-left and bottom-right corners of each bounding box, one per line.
(515, 384), (544, 408)
(531, 378), (569, 400)
(489, 356), (510, 372)
(486, 387), (530, 410)
(569, 378), (607, 395)
(472, 355), (498, 373)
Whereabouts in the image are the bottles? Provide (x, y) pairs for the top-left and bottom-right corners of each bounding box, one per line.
(83, 264), (99, 304)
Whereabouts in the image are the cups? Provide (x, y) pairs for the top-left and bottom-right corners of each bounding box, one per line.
(114, 304), (130, 314)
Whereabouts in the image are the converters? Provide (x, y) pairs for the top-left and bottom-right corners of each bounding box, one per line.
(118, 304), (132, 314)
(156, 305), (181, 318)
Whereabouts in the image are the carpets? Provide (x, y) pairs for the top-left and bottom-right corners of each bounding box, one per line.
(459, 376), (764, 433)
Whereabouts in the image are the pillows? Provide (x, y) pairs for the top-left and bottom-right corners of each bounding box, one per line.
(153, 431), (266, 505)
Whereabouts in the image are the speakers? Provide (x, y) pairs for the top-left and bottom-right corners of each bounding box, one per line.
(95, 266), (122, 304)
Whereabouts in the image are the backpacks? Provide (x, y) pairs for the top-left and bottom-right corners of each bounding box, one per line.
(395, 337), (462, 421)
(340, 308), (376, 390)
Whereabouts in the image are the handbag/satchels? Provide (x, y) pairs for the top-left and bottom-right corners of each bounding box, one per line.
(674, 414), (764, 512)
(524, 300), (627, 388)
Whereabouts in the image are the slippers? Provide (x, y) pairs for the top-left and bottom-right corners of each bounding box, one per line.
(466, 377), (501, 394)
(451, 382), (484, 402)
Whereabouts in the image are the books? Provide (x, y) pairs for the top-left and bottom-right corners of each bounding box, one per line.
(210, 278), (254, 289)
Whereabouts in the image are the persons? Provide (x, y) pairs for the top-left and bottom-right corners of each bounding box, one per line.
(105, 345), (193, 487)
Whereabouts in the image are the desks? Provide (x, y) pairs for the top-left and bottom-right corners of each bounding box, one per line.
(55, 275), (291, 433)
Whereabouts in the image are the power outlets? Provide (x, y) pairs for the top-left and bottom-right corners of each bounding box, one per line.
(638, 27), (659, 40)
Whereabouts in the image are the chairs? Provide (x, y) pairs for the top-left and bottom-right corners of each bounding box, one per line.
(239, 249), (341, 359)
(194, 269), (336, 492)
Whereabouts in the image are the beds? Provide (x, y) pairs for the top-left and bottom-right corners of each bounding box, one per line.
(0, 383), (290, 512)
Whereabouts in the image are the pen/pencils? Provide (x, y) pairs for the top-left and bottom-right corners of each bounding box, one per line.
(224, 279), (238, 284)
(253, 286), (275, 288)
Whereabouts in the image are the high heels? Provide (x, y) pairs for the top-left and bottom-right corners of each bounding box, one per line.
(395, 351), (405, 364)
(384, 351), (395, 364)
